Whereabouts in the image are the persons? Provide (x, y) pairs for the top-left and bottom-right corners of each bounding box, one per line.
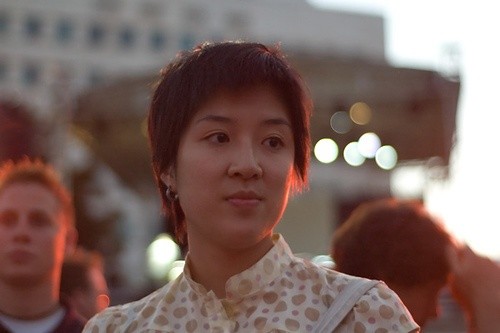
(55, 245), (110, 317)
(0, 157), (86, 333)
(313, 197), (500, 332)
(80, 41), (422, 333)
